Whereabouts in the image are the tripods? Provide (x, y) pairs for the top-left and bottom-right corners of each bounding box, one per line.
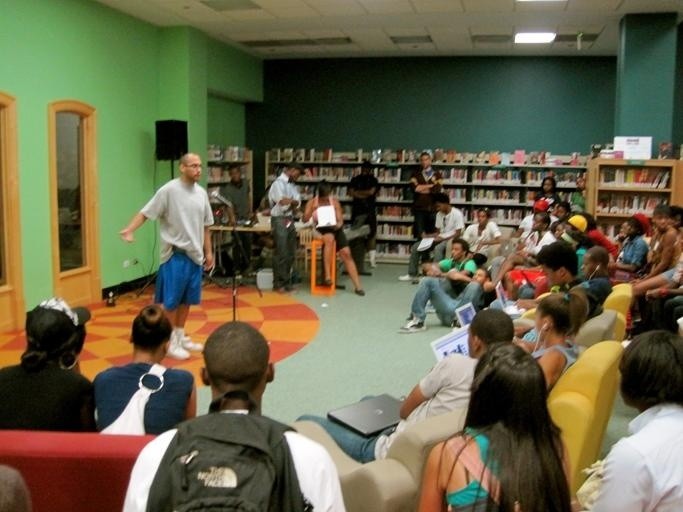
(136, 162), (226, 298)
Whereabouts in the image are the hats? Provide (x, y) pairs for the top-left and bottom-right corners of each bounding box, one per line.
(533, 200), (551, 214)
(23, 296), (91, 343)
(568, 214), (589, 232)
(286, 162), (306, 176)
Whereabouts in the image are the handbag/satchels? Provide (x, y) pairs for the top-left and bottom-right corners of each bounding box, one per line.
(103, 364), (167, 439)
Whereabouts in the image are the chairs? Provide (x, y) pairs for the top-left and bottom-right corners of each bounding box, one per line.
(520, 283), (635, 510)
(289, 407), (468, 511)
(0, 428), (163, 511)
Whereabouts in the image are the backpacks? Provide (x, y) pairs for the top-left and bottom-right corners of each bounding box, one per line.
(144, 391), (313, 511)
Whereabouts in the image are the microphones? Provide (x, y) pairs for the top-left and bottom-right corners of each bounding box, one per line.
(211, 191), (232, 208)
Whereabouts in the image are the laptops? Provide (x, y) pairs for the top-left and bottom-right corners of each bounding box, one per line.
(327, 393), (403, 439)
(430, 324), (471, 363)
(495, 280), (526, 315)
(455, 302), (476, 327)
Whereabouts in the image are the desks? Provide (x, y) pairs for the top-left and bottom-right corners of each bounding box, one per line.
(204, 219), (317, 285)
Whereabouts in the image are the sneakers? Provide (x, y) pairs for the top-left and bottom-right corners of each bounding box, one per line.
(277, 264), (378, 294)
(180, 337), (203, 351)
(164, 341), (190, 360)
(398, 313), (425, 333)
(398, 274), (420, 282)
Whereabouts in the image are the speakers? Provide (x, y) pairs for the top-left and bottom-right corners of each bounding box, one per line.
(155, 120), (188, 161)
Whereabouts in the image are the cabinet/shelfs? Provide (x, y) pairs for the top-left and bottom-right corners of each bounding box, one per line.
(588, 155), (683, 228)
(209, 148), (254, 221)
(263, 147), (588, 268)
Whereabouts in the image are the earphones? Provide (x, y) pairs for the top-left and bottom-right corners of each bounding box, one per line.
(596, 265), (599, 269)
(542, 323), (547, 328)
